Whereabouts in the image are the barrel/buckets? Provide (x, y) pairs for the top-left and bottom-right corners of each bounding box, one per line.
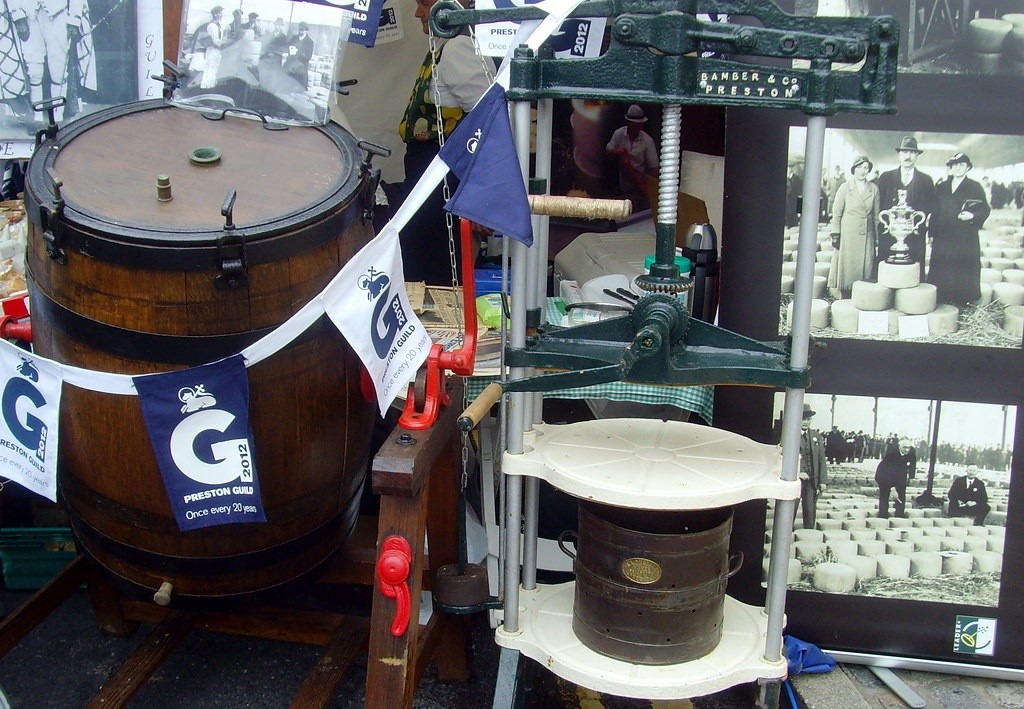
(554, 505), (746, 668)
(23, 99), (390, 611)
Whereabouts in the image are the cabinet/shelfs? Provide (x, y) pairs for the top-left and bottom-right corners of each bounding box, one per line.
(483, 17), (870, 701)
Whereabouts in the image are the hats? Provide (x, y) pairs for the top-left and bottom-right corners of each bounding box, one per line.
(896, 136), (923, 155)
(851, 156), (873, 175)
(624, 104), (649, 123)
(273, 18), (285, 27)
(298, 21), (310, 32)
(803, 404), (816, 417)
(250, 13), (259, 18)
(947, 153), (972, 171)
(66, 0), (83, 27)
(10, 0), (27, 22)
(211, 5), (225, 14)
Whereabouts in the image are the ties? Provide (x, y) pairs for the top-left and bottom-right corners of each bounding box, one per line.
(803, 432), (807, 443)
(969, 481), (972, 487)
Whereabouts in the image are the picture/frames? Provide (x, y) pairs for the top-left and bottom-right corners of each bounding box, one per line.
(172, 0), (346, 127)
(715, 67), (1024, 681)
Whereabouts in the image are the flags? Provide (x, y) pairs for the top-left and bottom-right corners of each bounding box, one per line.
(440, 82), (534, 249)
(319, 224), (435, 420)
(131, 353), (269, 534)
(0, 337), (65, 503)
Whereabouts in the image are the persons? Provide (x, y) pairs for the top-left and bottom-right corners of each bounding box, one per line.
(789, 402), (828, 531)
(10, 10), (55, 121)
(947, 463), (992, 526)
(878, 134), (938, 285)
(874, 436), (918, 519)
(811, 425), (1014, 472)
(824, 154), (882, 301)
(398, 0), (495, 285)
(604, 105), (660, 179)
(197, 6), (315, 119)
(922, 152), (992, 315)
